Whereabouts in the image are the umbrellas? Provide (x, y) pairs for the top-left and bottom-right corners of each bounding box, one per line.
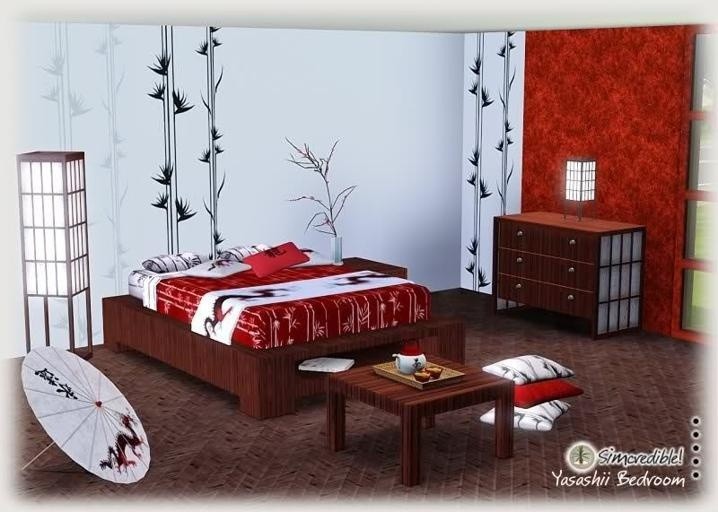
(20, 345), (150, 485)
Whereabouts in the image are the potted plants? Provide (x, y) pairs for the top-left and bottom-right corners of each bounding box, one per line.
(279, 133), (357, 266)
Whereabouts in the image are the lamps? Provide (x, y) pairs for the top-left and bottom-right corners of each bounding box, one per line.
(13, 150), (93, 365)
(562, 158), (597, 224)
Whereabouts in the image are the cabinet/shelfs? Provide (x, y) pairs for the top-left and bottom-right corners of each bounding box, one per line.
(490, 210), (648, 343)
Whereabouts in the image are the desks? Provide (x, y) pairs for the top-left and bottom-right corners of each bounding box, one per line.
(323, 350), (515, 487)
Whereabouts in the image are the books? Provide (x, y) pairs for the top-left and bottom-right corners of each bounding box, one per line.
(298, 356), (355, 373)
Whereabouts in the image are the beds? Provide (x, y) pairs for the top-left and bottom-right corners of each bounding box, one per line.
(101, 252), (468, 423)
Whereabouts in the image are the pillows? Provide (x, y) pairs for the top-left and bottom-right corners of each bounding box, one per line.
(479, 352), (576, 386)
(478, 399), (575, 432)
(180, 258), (253, 280)
(509, 377), (584, 410)
(140, 252), (208, 274)
(241, 242), (309, 280)
(216, 243), (271, 263)
(285, 246), (332, 268)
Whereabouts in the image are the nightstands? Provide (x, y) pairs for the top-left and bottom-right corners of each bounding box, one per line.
(340, 256), (408, 280)
